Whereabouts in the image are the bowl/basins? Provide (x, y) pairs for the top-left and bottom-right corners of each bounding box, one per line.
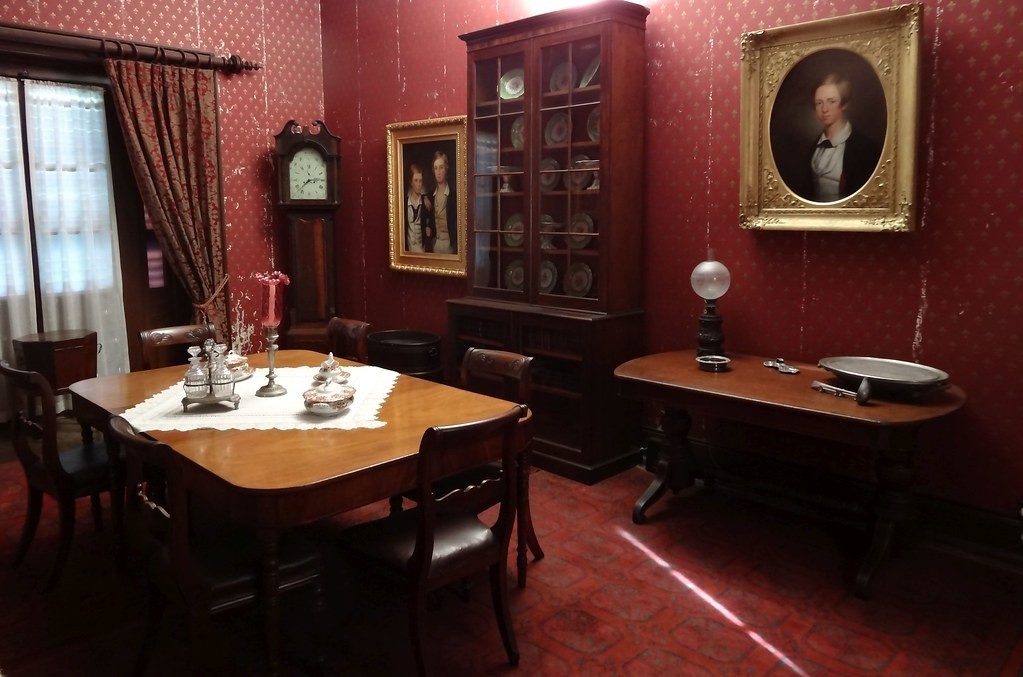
(696, 354), (731, 372)
(365, 330), (441, 372)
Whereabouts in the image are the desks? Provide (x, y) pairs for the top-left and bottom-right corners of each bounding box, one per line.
(68, 341), (536, 677)
(9, 330), (97, 436)
(615, 344), (968, 596)
(445, 297), (640, 483)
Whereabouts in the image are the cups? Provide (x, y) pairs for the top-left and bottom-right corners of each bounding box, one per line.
(318, 351), (343, 379)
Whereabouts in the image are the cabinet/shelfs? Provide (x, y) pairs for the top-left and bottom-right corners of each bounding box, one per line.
(455, 0), (650, 315)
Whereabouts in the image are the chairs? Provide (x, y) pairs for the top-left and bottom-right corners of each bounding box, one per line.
(0, 323), (545, 677)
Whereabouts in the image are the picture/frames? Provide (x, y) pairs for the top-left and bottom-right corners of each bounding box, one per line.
(385, 115), (467, 279)
(737, 1), (926, 236)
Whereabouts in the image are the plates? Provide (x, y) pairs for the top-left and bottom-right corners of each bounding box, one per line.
(539, 261), (557, 292)
(564, 213), (594, 248)
(312, 371), (350, 384)
(537, 214), (556, 242)
(504, 259), (524, 291)
(496, 54), (601, 99)
(510, 105), (605, 148)
(564, 154), (592, 189)
(539, 157), (560, 191)
(504, 212), (525, 246)
(562, 261), (592, 297)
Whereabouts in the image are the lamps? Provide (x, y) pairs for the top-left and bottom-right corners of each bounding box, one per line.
(690, 262), (730, 358)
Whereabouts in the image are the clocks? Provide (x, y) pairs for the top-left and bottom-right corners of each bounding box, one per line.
(275, 119), (345, 214)
